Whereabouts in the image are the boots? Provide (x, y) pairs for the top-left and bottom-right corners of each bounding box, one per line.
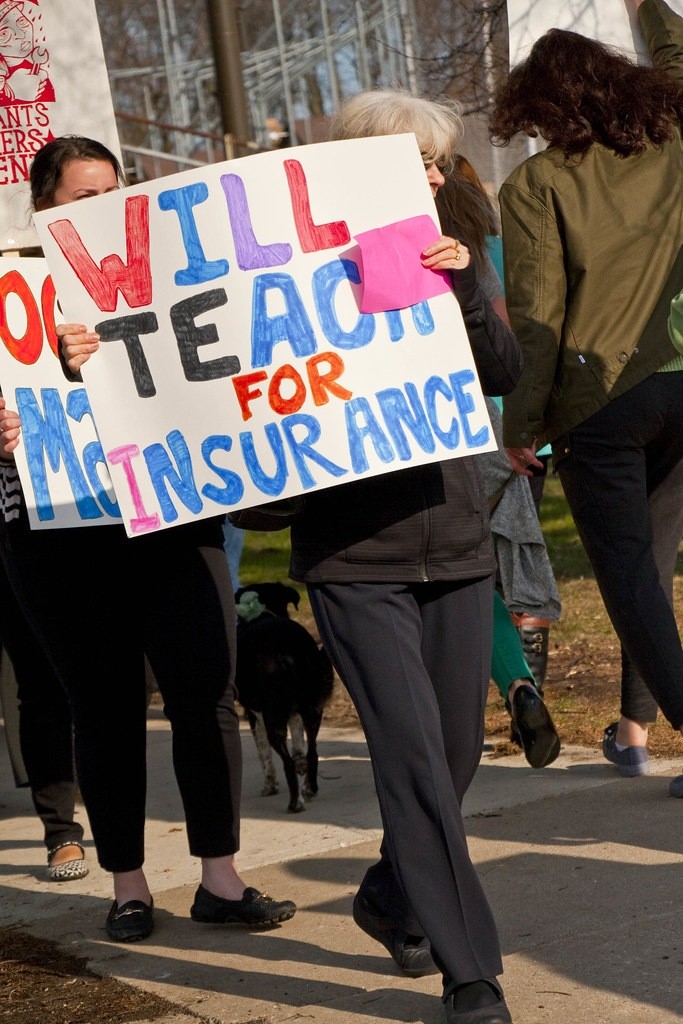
(506, 611), (550, 748)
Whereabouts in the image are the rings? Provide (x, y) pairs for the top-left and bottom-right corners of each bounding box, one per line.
(455, 250), (460, 259)
(0, 428), (4, 433)
(454, 240), (460, 249)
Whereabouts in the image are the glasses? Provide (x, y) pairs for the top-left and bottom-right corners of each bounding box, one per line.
(421, 152), (446, 176)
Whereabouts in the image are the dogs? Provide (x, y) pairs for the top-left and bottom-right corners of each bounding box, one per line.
(235, 583), (335, 811)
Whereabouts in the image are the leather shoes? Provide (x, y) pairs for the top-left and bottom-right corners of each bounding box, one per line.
(440, 977), (512, 1024)
(353, 911), (442, 975)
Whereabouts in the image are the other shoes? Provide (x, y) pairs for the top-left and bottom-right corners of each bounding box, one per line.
(106, 895), (154, 943)
(602, 723), (649, 777)
(508, 679), (561, 769)
(47, 841), (89, 880)
(670, 775), (683, 798)
(190, 883), (297, 927)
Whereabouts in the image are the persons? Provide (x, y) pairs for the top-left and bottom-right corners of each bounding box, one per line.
(52, 88), (524, 1024)
(1, 391), (109, 881)
(447, 151), (563, 767)
(491, 27), (683, 800)
(0, 133), (296, 946)
